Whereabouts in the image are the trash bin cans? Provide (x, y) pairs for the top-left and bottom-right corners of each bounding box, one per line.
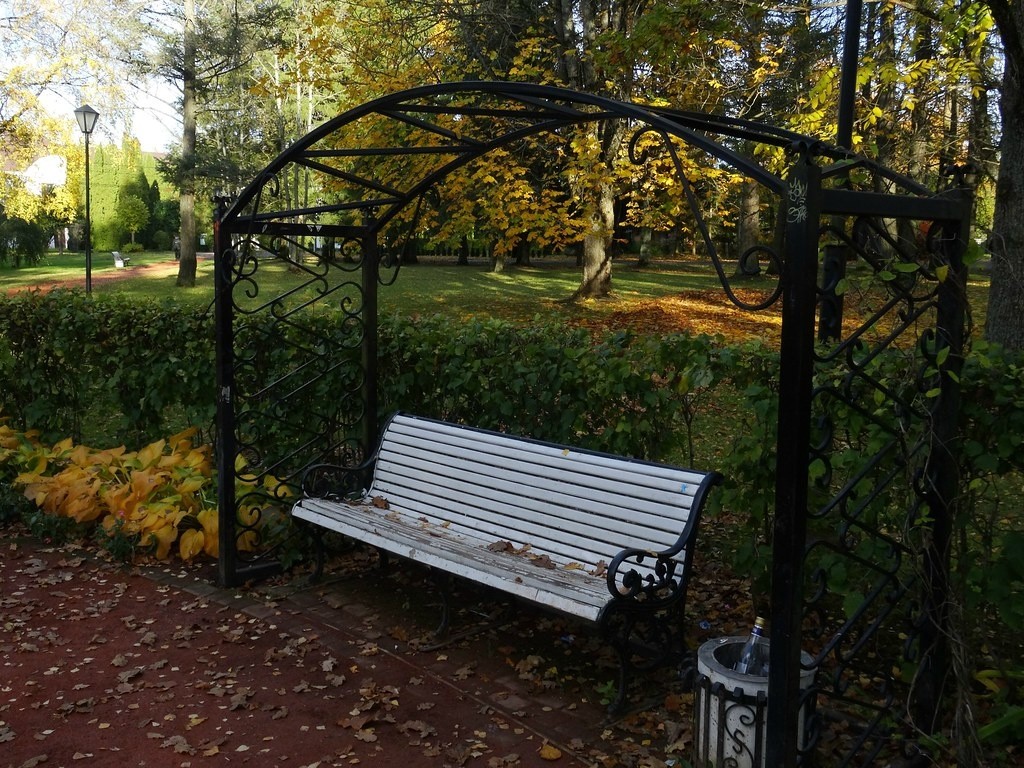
(682, 635), (819, 768)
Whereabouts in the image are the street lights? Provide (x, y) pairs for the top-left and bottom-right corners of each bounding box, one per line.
(72, 104), (100, 297)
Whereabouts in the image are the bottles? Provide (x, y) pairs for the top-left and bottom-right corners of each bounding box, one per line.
(731, 615), (766, 675)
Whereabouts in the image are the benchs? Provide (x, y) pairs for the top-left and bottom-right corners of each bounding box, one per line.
(291, 408), (724, 717)
(112, 252), (130, 268)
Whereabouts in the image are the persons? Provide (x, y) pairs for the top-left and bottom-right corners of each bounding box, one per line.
(173, 235), (182, 260)
